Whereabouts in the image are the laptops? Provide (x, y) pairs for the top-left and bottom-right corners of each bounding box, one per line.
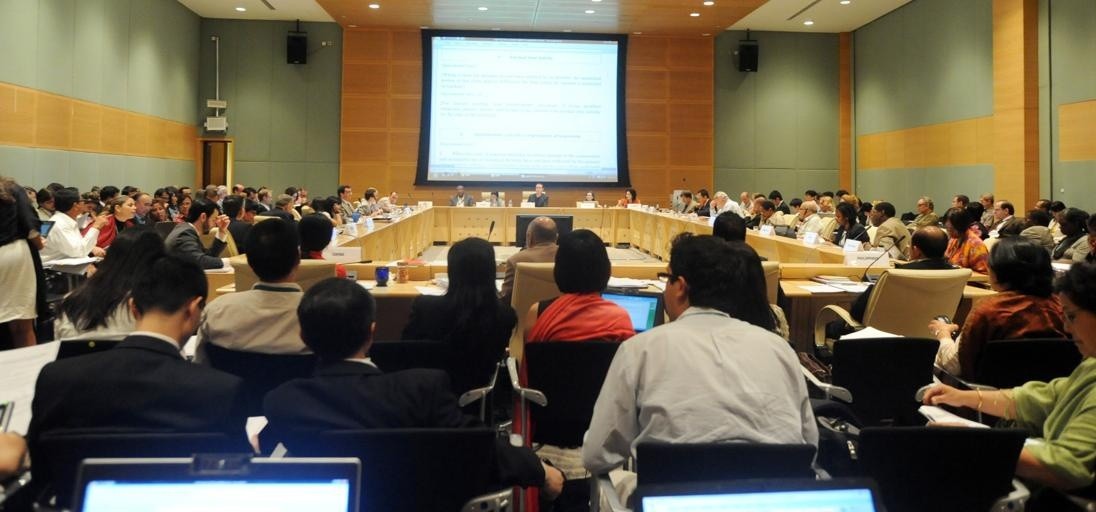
(373, 213), (393, 222)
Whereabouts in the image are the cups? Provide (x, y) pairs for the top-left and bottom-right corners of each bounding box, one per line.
(375, 266), (389, 287)
(352, 213), (360, 222)
(753, 225), (758, 234)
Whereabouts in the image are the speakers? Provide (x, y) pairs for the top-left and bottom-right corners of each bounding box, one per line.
(739, 40), (758, 73)
(286, 30), (308, 65)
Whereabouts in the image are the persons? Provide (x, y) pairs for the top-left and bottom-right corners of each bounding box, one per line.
(2, 182), (1095, 512)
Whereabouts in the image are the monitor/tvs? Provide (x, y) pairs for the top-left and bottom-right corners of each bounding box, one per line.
(600, 288), (664, 329)
(40, 221), (56, 236)
(71, 457), (361, 512)
(636, 480), (880, 512)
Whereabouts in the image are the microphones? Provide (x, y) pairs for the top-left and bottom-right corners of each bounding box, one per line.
(797, 208), (807, 247)
(356, 197), (369, 217)
(674, 195), (684, 216)
(339, 231), (364, 262)
(480, 219), (502, 244)
(334, 259), (373, 269)
(861, 234), (907, 282)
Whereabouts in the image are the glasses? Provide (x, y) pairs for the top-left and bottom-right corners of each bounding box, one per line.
(1057, 306), (1083, 326)
(196, 309), (209, 329)
(654, 271), (671, 285)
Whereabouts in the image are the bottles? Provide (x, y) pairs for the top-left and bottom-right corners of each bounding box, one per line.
(877, 244), (885, 251)
(603, 200), (608, 208)
(397, 261), (409, 284)
(508, 200), (512, 207)
(656, 202), (660, 210)
